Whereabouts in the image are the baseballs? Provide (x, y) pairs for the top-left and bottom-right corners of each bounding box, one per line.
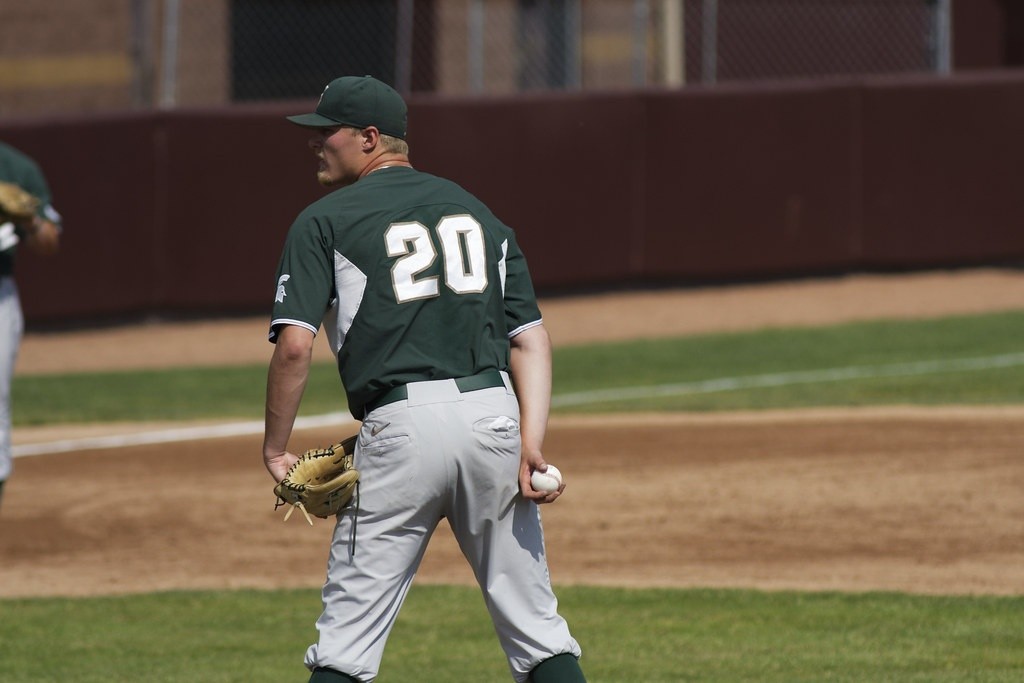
(531, 463), (562, 495)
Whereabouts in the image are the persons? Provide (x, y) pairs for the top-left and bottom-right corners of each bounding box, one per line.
(0, 142), (63, 495)
(262, 74), (588, 683)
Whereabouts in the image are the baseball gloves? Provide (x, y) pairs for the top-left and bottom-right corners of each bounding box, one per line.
(0, 180), (47, 225)
(272, 434), (361, 521)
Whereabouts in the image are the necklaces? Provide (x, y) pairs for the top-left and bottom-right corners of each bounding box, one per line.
(365, 164), (413, 177)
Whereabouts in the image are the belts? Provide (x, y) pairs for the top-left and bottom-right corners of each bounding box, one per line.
(366, 371), (505, 413)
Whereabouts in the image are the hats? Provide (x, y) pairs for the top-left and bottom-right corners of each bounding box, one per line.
(286, 74), (408, 140)
